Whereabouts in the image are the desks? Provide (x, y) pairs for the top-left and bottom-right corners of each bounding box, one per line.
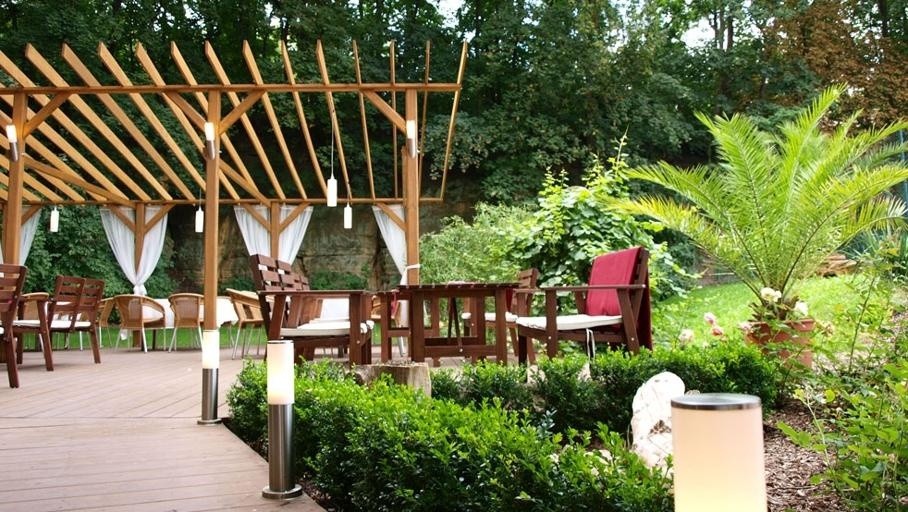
(396, 283), (520, 365)
(375, 291), (441, 367)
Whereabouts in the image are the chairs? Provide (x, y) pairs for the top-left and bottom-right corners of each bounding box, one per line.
(0, 264), (203, 388)
(512, 246), (652, 382)
(461, 267), (538, 364)
(226, 254), (374, 369)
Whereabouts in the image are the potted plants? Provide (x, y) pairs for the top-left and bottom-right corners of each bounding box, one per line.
(589, 80), (908, 374)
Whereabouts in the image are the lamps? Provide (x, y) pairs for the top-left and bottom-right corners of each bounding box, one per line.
(343, 181), (352, 229)
(50, 200), (60, 232)
(6, 125), (19, 162)
(327, 116), (338, 207)
(406, 119), (416, 159)
(195, 186), (205, 233)
(204, 122), (216, 161)
